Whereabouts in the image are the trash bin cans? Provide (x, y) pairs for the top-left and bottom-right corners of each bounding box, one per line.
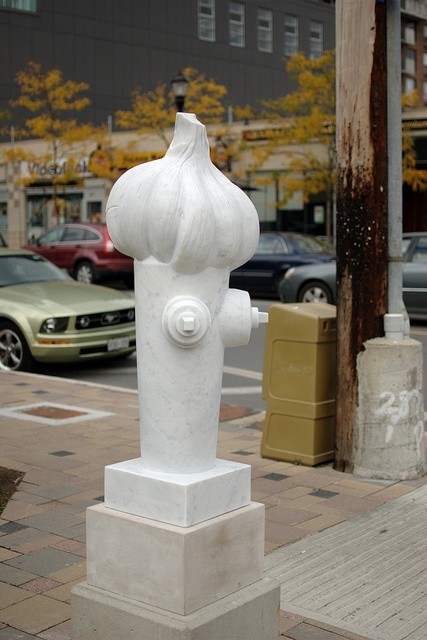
(259, 302), (337, 467)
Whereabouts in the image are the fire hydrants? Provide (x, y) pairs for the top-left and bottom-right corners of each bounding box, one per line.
(104, 110), (270, 529)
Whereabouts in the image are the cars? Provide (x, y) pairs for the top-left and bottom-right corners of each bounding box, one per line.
(229, 229), (337, 299)
(279, 232), (427, 321)
(0, 246), (137, 372)
(18, 221), (133, 284)
